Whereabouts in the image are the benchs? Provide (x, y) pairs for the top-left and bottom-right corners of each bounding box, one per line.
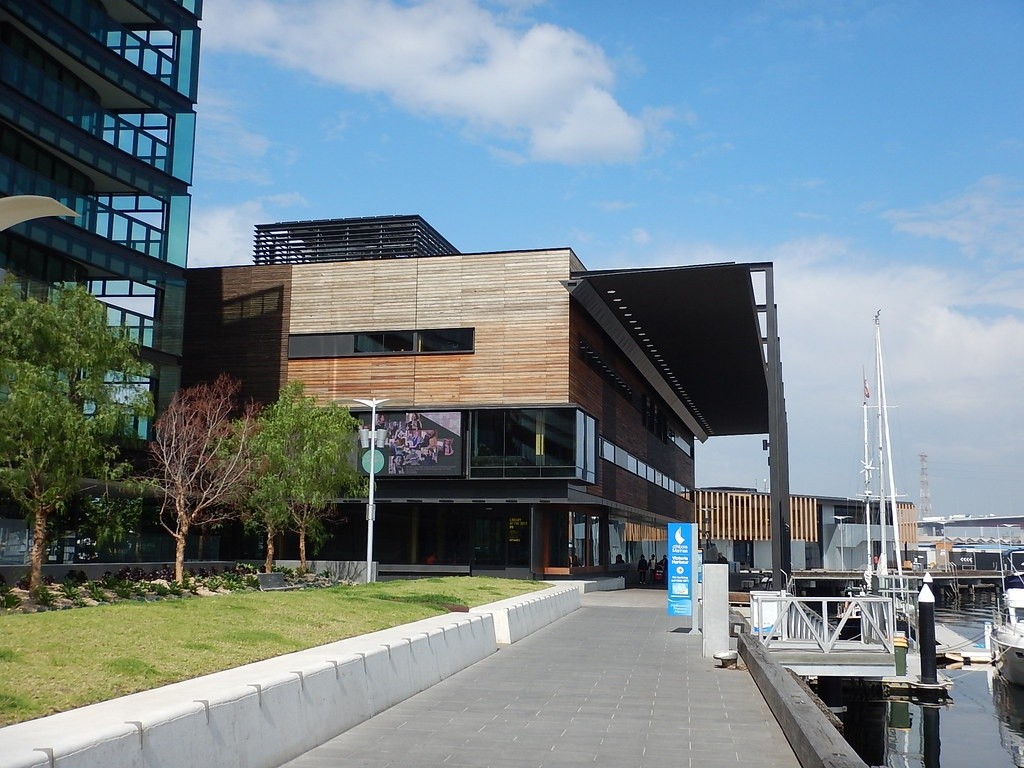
(543, 568), (574, 576)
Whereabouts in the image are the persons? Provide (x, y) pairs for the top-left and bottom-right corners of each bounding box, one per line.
(637, 553), (648, 585)
(377, 413), (454, 474)
(874, 554), (878, 571)
(616, 554), (624, 563)
(647, 554), (658, 583)
(660, 554), (668, 585)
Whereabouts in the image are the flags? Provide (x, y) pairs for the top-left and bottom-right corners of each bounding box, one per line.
(864, 377), (869, 398)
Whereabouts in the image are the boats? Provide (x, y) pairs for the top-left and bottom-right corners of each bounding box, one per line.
(986, 587), (1024, 690)
